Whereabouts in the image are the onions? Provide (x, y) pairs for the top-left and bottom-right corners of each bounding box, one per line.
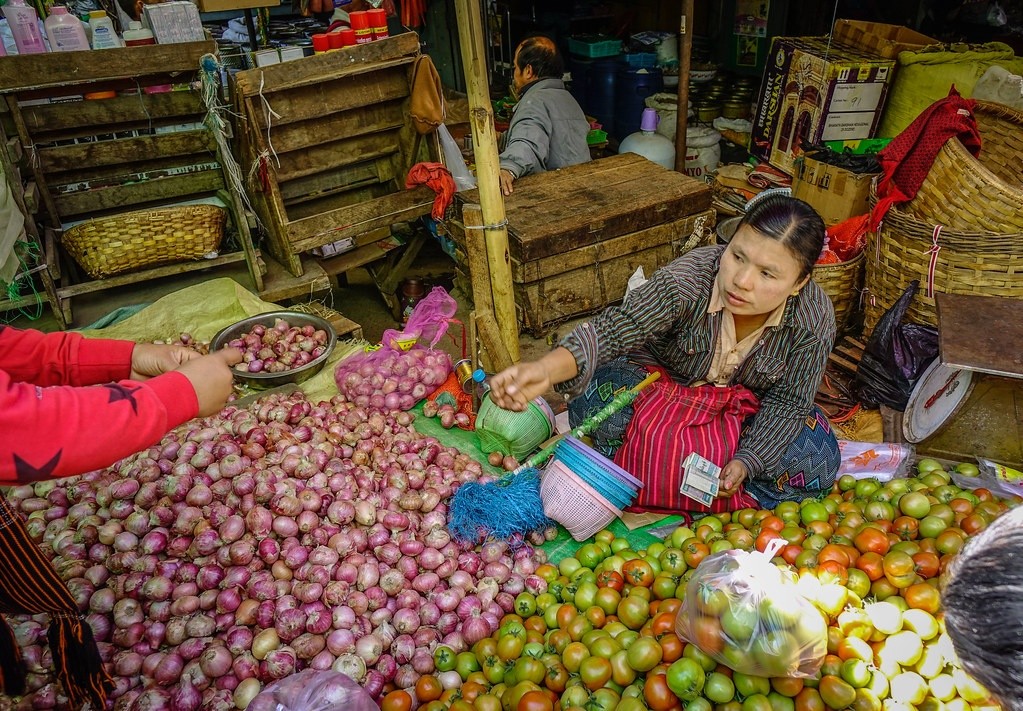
(0, 316), (558, 711)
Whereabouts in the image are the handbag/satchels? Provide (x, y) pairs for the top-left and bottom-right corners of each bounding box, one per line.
(246, 668), (380, 711)
(674, 539), (828, 680)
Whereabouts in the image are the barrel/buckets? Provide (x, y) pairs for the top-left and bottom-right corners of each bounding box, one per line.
(587, 60), (620, 138)
(613, 67), (663, 143)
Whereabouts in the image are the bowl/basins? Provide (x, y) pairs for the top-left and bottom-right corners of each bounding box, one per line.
(687, 81), (755, 123)
(208, 310), (337, 392)
(688, 69), (718, 83)
(663, 75), (679, 85)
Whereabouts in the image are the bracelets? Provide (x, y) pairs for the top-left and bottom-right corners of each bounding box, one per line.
(508, 170), (517, 179)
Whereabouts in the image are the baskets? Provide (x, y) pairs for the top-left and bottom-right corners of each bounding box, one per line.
(811, 99), (1023, 342)
(567, 37), (623, 58)
(475, 388), (556, 462)
(586, 122), (607, 144)
(540, 434), (645, 542)
(60, 204), (229, 280)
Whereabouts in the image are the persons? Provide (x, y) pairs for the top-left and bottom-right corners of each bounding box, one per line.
(0, 324), (243, 487)
(491, 195), (841, 530)
(499, 36), (594, 195)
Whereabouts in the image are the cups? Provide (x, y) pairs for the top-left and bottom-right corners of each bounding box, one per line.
(639, 107), (660, 131)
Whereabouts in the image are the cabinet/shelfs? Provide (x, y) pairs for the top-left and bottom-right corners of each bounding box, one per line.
(232, 33), (451, 279)
(1, 40), (263, 327)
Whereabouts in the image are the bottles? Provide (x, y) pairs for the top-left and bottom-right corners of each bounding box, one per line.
(122, 21), (157, 47)
(618, 130), (675, 170)
(311, 8), (389, 56)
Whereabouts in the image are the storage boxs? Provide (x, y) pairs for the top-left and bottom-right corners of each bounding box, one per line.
(834, 20), (941, 59)
(439, 152), (714, 341)
(568, 37), (623, 60)
(747, 35), (893, 176)
(791, 151), (874, 229)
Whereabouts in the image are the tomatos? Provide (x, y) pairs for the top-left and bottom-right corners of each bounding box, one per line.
(380, 458), (1023, 710)
